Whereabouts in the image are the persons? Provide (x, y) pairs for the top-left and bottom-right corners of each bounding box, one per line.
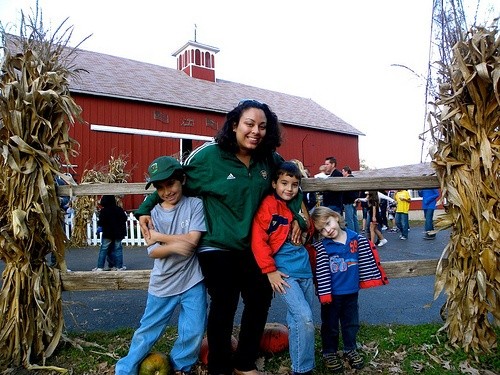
(288, 158), (316, 213)
(311, 206), (388, 370)
(135, 100), (307, 375)
(394, 189), (412, 240)
(45, 168), (71, 268)
(354, 190), (388, 248)
(418, 188), (439, 240)
(91, 194), (128, 271)
(323, 157), (344, 216)
(341, 166), (359, 233)
(252, 162), (317, 375)
(359, 190), (399, 231)
(313, 164), (332, 178)
(114, 157), (207, 375)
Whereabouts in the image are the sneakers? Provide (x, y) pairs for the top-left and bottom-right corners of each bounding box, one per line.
(344, 349), (364, 369)
(321, 354), (344, 373)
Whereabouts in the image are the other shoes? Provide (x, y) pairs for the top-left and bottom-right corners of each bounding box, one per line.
(378, 238), (387, 246)
(117, 266), (126, 271)
(92, 267), (102, 272)
(104, 267), (116, 271)
(401, 237), (406, 240)
(423, 236), (436, 240)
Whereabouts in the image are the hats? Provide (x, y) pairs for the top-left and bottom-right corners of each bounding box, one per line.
(144, 156), (184, 190)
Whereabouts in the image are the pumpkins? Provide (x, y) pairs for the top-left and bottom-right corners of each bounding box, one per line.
(136, 351), (172, 374)
(259, 323), (289, 353)
(200, 333), (238, 365)
